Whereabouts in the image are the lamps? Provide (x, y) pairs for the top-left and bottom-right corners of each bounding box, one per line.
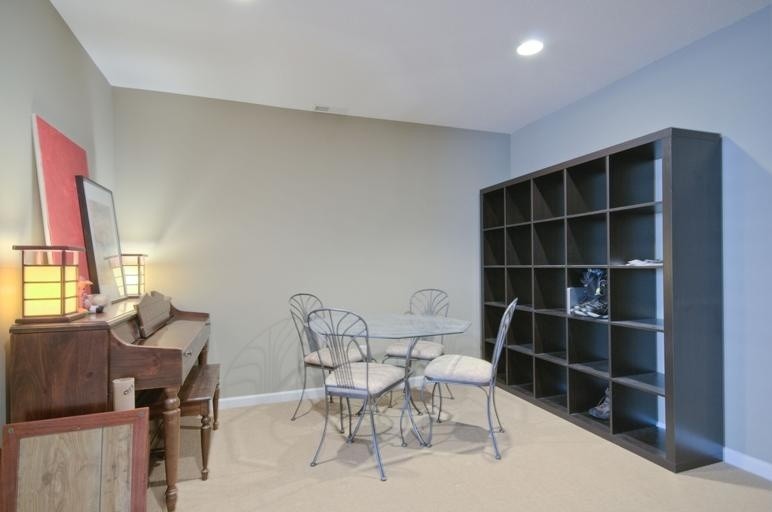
(13, 245), (86, 323)
(122, 253), (149, 297)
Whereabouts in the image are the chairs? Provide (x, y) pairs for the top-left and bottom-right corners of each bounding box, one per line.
(289, 291), (374, 432)
(308, 308), (407, 481)
(422, 297), (519, 459)
(382, 289), (456, 412)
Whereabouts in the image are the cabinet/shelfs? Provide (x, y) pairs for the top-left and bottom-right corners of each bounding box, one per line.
(479, 128), (724, 473)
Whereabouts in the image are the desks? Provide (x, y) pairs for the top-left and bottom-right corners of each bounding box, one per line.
(303, 315), (472, 447)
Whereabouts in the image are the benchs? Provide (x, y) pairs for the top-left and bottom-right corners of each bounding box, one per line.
(147, 362), (221, 480)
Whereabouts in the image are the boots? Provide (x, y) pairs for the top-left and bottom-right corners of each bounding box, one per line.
(571, 269), (608, 319)
(589, 387), (609, 422)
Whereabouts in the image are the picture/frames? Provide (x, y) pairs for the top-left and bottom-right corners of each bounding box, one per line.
(0, 407), (150, 512)
(76, 175), (127, 306)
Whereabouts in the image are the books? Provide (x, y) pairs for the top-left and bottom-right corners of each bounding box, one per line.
(624, 259), (663, 267)
(566, 286), (594, 316)
